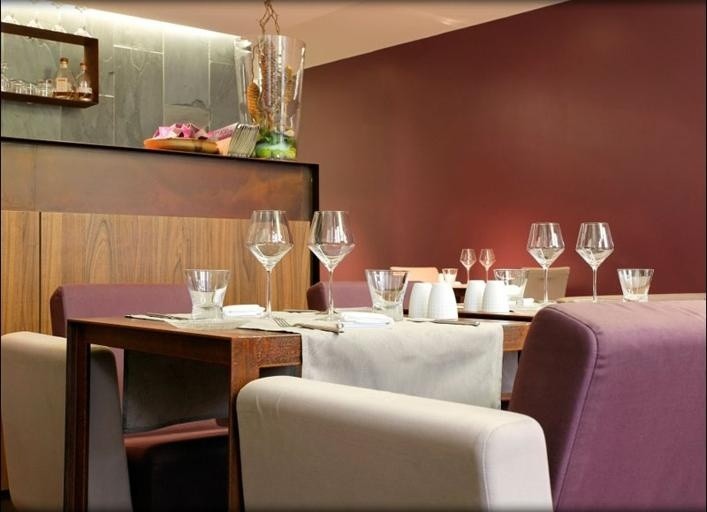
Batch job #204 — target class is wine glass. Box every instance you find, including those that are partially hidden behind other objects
[248,209,294,320]
[307,209,356,320]
[478,249,496,284]
[575,222,614,302]
[527,222,565,308]
[459,248,476,284]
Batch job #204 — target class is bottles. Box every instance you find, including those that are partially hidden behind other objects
[53,57,78,101]
[75,62,92,103]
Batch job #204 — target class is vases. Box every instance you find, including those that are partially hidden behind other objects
[231,34,307,162]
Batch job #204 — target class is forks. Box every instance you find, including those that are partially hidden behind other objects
[272,317,340,334]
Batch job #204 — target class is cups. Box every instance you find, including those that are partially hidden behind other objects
[0,64,53,99]
[365,268,407,321]
[409,267,528,322]
[184,267,230,326]
[615,267,654,304]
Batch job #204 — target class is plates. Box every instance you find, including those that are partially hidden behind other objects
[144,138,220,154]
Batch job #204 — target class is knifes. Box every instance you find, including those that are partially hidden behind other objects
[146,312,191,321]
[409,318,480,327]
